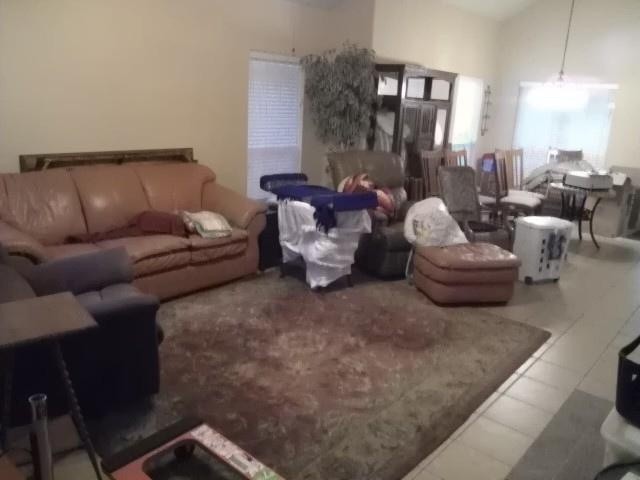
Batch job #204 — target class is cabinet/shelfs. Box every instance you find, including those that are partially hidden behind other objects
[369,64,460,178]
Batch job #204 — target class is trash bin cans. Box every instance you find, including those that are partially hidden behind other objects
[512,215,575,285]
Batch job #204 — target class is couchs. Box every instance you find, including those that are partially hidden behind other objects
[0,160,270,308]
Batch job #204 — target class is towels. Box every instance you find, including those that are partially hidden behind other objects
[66,210,232,244]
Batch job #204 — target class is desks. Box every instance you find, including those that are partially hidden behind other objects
[0,289,106,478]
[277,201,368,291]
[549,180,616,251]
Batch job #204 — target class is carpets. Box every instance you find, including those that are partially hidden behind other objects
[72,262,552,479]
[498,389,616,480]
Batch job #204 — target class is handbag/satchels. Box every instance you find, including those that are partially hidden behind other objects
[185,207,232,239]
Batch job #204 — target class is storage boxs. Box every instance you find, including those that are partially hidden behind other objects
[598,336,640,468]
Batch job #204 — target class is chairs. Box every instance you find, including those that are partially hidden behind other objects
[0,241,164,409]
[321,151,417,283]
[421,147,546,247]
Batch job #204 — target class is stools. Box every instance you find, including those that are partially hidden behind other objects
[412,243,521,308]
